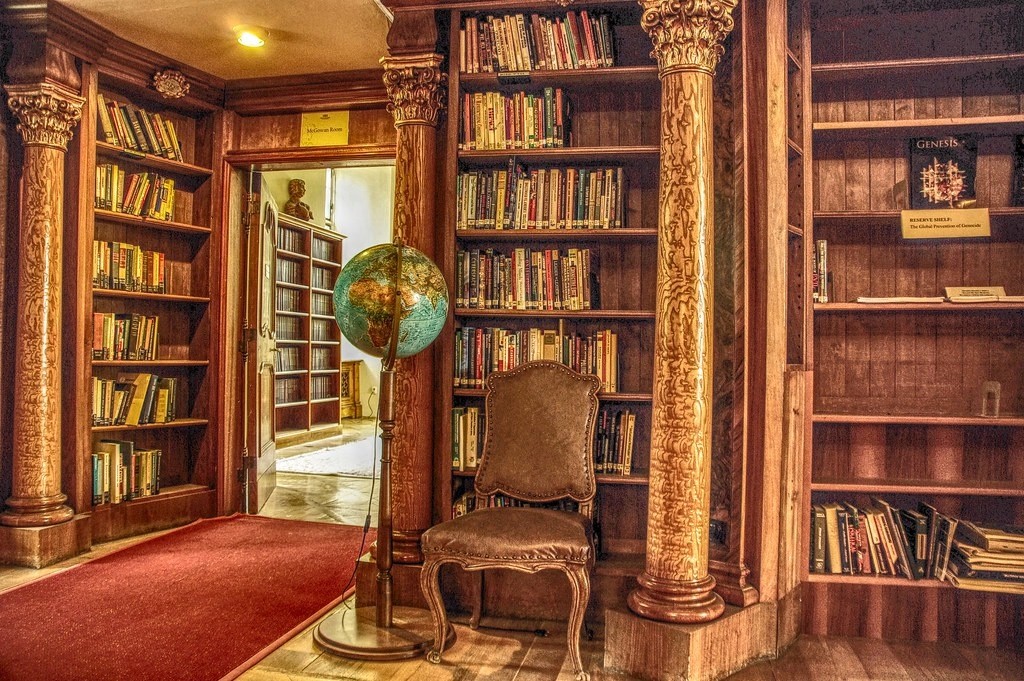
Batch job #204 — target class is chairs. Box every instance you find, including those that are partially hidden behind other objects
[416,359,605,681]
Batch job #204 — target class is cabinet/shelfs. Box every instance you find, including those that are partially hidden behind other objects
[797,1,1022,657]
[439,4,662,563]
[74,21,227,546]
[274,210,349,450]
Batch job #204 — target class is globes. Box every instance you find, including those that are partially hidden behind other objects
[314,237,457,662]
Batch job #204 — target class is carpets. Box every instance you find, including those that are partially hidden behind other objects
[273,433,380,480]
[0,508,377,681]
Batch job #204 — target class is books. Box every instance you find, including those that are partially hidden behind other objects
[806,497,1024,600]
[845,294,1024,303]
[86,87,184,505]
[907,134,980,211]
[815,238,831,304]
[811,241,820,305]
[456,6,640,557]
[267,220,345,412]
